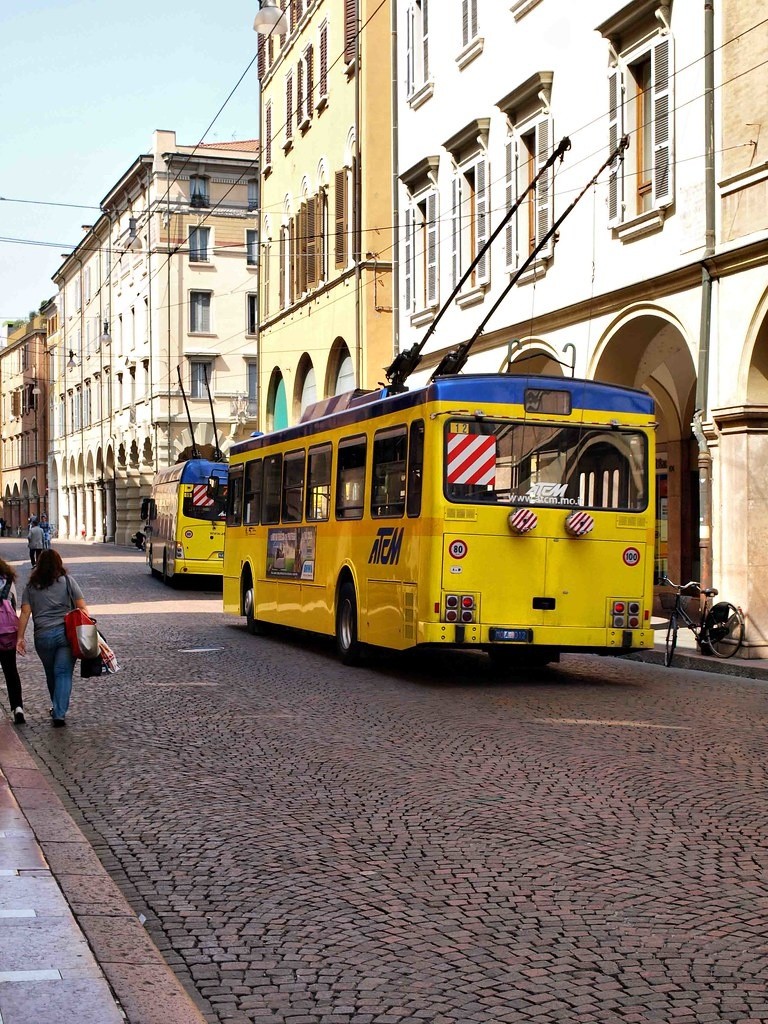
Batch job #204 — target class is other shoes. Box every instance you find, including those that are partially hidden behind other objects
[54,719,66,728]
[50,707,54,717]
[14,706,26,724]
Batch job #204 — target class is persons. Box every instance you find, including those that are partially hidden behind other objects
[131,531,146,552]
[15,548,89,726]
[0,557,25,724]
[0,513,52,569]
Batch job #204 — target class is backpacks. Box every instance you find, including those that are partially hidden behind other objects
[0,577,21,650]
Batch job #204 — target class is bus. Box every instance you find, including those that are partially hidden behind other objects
[141,364,229,592]
[220,138,659,674]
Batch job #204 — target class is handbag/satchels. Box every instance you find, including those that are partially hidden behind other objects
[64,574,102,659]
[80,630,121,678]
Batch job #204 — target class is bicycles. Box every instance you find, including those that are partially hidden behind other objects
[658,576,746,667]
